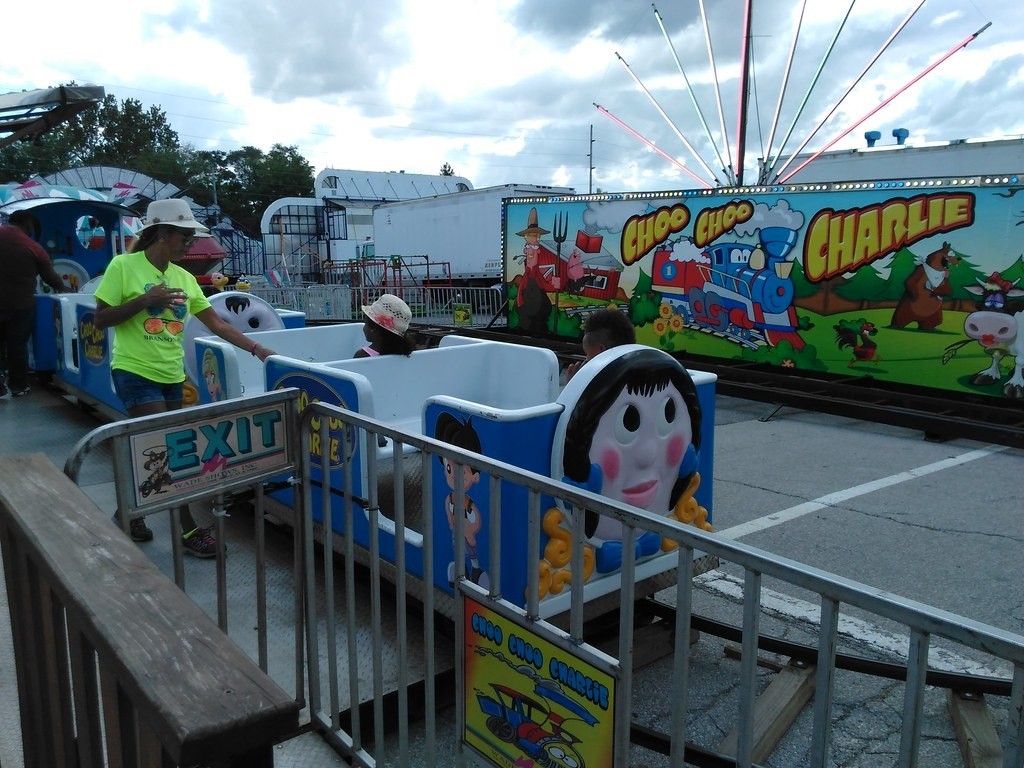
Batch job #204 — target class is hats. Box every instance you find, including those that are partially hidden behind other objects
[361,293,413,339]
[134,198,210,233]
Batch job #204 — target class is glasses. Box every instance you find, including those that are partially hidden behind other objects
[171,228,198,247]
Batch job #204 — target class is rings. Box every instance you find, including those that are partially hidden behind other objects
[170,299,175,305]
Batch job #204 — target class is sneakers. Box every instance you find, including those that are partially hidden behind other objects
[112,508,153,541]
[12,384,33,395]
[0,372,8,396]
[181,526,228,558]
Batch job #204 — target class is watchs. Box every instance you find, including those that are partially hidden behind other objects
[248,342,259,356]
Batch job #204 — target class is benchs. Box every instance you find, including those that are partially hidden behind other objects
[202,313,368,403]
[421,360,719,543]
[318,342,552,507]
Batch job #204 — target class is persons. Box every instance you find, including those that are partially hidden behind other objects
[566,308,638,385]
[352,294,417,360]
[93,197,280,560]
[0,209,77,398]
